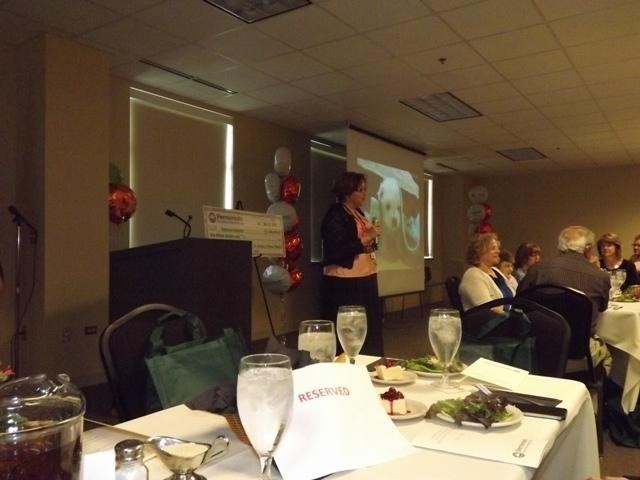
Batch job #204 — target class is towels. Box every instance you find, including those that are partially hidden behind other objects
[184,334,312,414]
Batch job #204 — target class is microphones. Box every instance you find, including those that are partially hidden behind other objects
[165,208,193,239]
[372,216,381,244]
[7,204,38,234]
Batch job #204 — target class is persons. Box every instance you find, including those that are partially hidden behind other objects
[457,222,639,429]
[318,168,384,354]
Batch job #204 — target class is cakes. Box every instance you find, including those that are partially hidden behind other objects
[379,386,407,416]
[376,357,406,381]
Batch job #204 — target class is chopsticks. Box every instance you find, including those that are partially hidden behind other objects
[476,383,492,397]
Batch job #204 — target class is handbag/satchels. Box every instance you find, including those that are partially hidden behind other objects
[604,397,640,448]
[458,338,540,373]
[143,328,251,410]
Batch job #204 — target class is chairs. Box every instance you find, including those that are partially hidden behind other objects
[99,304,208,423]
[445,269,604,458]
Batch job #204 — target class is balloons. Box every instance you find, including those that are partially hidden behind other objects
[105,184,140,225]
[109,162,125,185]
[256,146,305,299]
[466,183,493,234]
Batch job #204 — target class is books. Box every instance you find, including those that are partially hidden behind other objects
[411,415,560,471]
[77,416,161,470]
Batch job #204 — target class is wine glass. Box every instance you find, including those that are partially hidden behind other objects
[335,305,368,366]
[236,352,294,480]
[429,308,462,389]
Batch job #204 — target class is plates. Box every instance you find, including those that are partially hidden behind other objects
[416,362,467,377]
[385,399,428,420]
[436,404,524,428]
[370,371,417,385]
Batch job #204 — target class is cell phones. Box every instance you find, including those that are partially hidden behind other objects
[512,402,567,420]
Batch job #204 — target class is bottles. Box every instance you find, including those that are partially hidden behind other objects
[114,440,149,479]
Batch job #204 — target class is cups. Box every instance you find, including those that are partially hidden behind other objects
[297,320,337,363]
[631,285,640,299]
[608,269,627,299]
[144,434,230,479]
[0,393,87,480]
